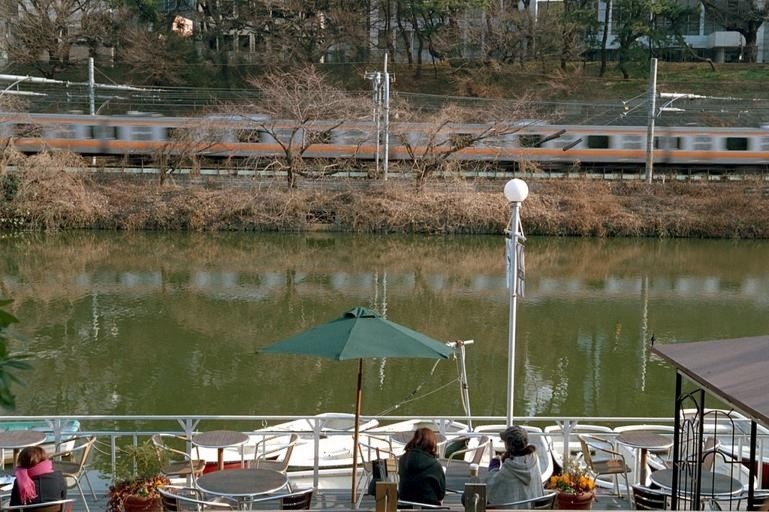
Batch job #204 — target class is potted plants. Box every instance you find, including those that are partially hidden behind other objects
[93,433,178,511]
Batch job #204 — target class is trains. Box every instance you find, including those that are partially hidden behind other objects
[0,107,769,179]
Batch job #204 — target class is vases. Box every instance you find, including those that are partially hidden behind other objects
[554,491,594,510]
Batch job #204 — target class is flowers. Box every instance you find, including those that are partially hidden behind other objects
[548,451,598,492]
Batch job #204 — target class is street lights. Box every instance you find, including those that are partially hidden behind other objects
[500,176,532,426]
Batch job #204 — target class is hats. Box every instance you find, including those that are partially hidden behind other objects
[500,427,528,448]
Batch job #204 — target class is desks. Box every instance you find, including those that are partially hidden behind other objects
[649,469,745,511]
[436,463,490,495]
[191,432,250,472]
[615,432,675,487]
[390,432,448,448]
[0,431,46,476]
[194,468,289,510]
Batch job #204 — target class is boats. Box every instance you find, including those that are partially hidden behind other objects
[183,412,380,476]
[463,424,555,489]
[544,424,653,489]
[275,418,474,490]
[0,419,83,465]
[613,407,769,494]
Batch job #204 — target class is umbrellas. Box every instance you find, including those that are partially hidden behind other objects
[252,304,460,504]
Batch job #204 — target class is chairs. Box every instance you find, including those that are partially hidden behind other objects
[156,485,241,512]
[436,433,490,504]
[246,433,298,509]
[708,498,722,511]
[577,434,634,511]
[486,489,557,510]
[736,489,768,511]
[152,432,207,487]
[2,498,77,512]
[665,434,720,511]
[352,432,399,510]
[42,433,97,511]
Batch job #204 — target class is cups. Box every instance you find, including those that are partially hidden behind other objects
[468,463,479,480]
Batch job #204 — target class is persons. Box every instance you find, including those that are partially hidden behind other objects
[398,425,447,511]
[8,445,67,512]
[461,425,546,509]
